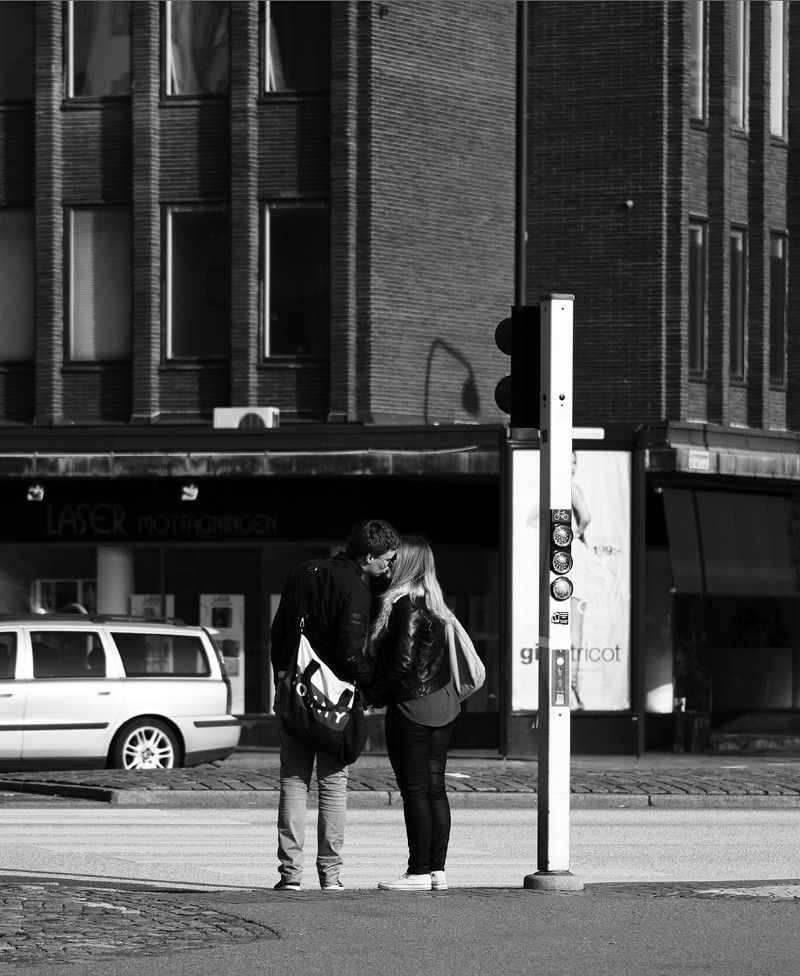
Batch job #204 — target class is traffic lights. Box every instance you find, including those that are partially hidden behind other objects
[492,302,538,430]
[551,509,574,601]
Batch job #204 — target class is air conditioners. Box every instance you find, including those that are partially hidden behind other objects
[214,407,280,428]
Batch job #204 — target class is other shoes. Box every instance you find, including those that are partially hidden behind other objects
[322,880,345,890]
[378,873,432,891]
[430,871,447,890]
[274,879,300,890]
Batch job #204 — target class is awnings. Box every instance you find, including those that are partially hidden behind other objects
[654,486,800,599]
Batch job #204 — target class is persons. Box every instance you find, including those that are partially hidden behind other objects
[269,521,402,889]
[570,452,592,708]
[364,536,461,891]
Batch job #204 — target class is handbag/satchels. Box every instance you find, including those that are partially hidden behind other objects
[279,634,368,766]
[445,618,486,702]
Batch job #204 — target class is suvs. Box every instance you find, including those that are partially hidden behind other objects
[1,614,241,772]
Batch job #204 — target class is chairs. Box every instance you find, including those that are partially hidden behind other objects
[87,648,103,675]
